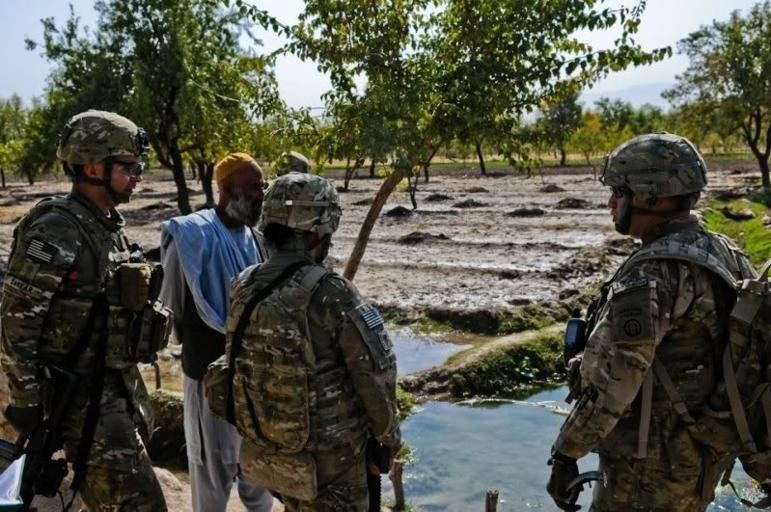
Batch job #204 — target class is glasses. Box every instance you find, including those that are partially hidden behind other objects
[112,159,142,176]
[613,187,631,198]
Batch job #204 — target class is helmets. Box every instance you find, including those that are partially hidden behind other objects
[275,150,308,174]
[56,110,145,166]
[600,132,708,201]
[261,171,342,238]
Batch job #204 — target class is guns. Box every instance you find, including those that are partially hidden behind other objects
[365,438,391,512]
[20,360,82,512]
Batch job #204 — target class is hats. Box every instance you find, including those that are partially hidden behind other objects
[216,153,254,183]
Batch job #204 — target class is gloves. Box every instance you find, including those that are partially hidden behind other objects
[548,453,585,511]
[5,406,43,438]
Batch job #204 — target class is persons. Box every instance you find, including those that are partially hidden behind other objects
[158,153,274,512]
[0,109,171,512]
[206,171,401,512]
[272,150,309,178]
[546,134,760,512]
